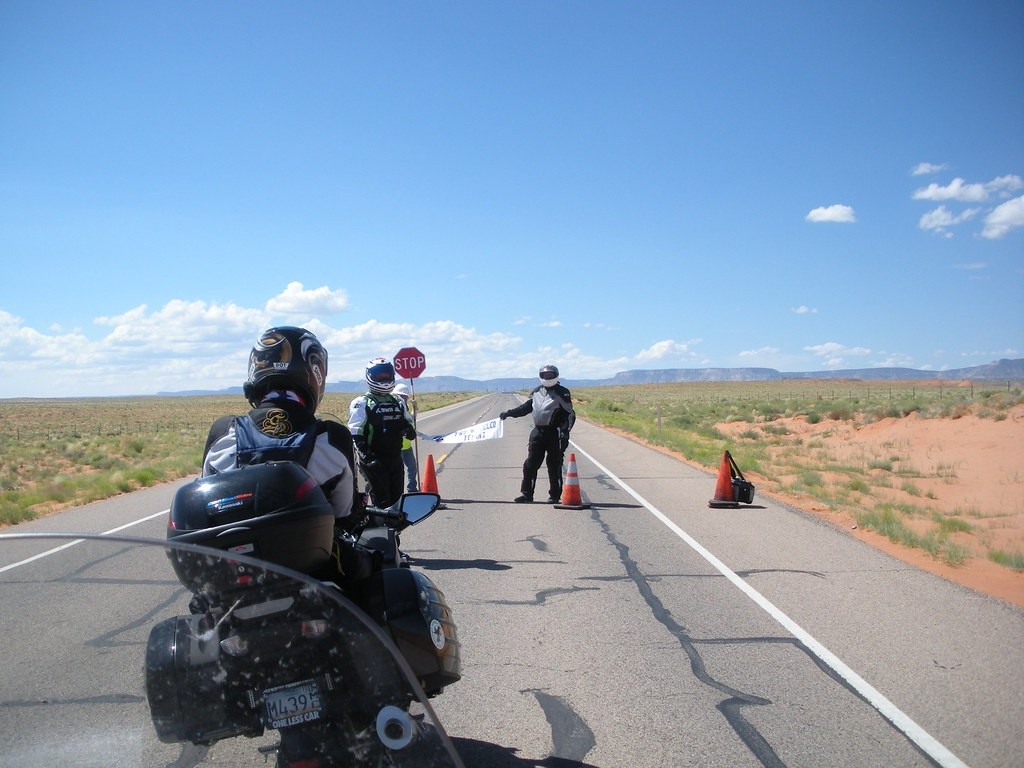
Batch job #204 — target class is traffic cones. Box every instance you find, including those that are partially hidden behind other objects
[422,454,447,510]
[552,453,591,511]
[707,449,740,508]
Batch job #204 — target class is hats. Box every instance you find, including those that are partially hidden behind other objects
[391,384,410,397]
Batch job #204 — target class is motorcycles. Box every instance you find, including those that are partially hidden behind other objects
[141,413,463,768]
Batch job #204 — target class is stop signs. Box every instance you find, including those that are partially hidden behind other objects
[393,347,426,379]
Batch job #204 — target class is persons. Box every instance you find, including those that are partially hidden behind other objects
[500,365,576,504]
[189,327,386,615]
[347,358,418,563]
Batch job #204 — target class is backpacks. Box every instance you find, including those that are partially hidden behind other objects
[235,415,322,469]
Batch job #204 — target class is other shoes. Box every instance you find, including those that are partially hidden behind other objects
[514,496,533,504]
[547,496,559,503]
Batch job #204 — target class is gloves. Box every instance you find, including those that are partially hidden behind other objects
[364,458,382,486]
[499,411,509,420]
[432,435,443,441]
[406,427,416,440]
[561,438,569,452]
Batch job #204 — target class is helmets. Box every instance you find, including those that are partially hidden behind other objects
[243,326,328,415]
[539,365,559,387]
[365,358,396,395]
